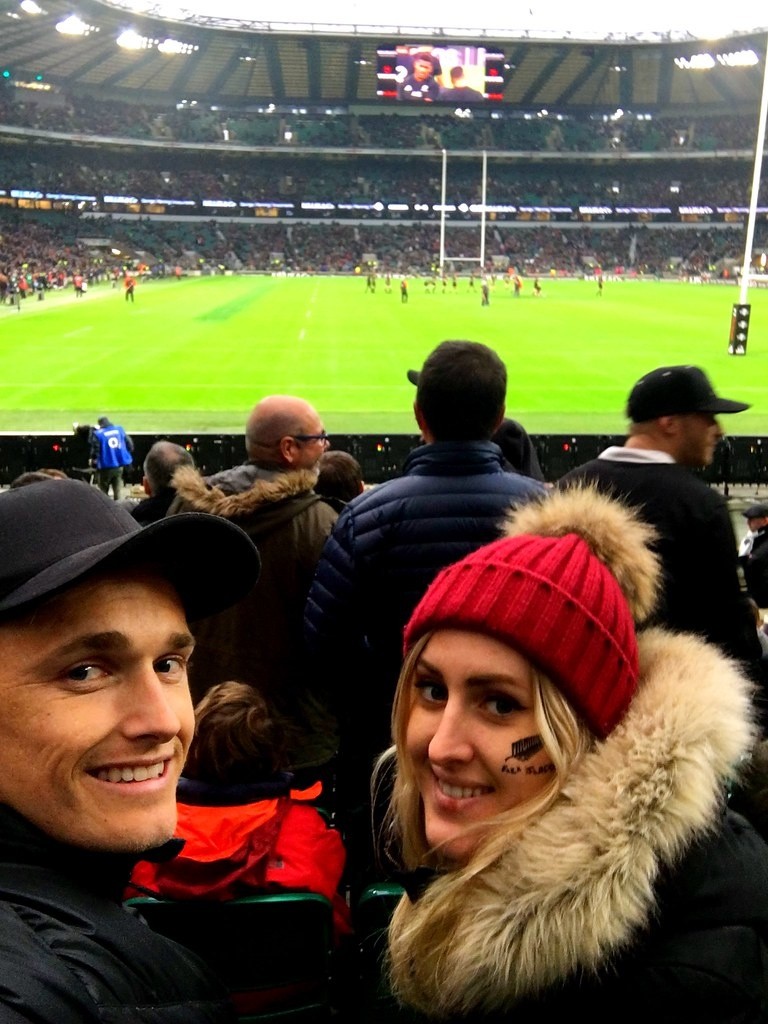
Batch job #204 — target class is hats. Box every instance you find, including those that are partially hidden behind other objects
[0,480,261,623]
[742,506,768,519]
[627,366,748,417]
[401,475,666,739]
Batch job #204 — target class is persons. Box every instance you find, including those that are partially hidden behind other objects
[401,281,408,302]
[0,340,768,905]
[124,272,136,301]
[0,68,767,304]
[373,479,768,1024]
[0,481,261,1024]
[400,55,485,101]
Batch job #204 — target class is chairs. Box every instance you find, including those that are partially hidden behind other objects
[121,881,433,1024]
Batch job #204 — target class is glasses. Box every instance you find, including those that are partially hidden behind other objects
[247,430,328,449]
